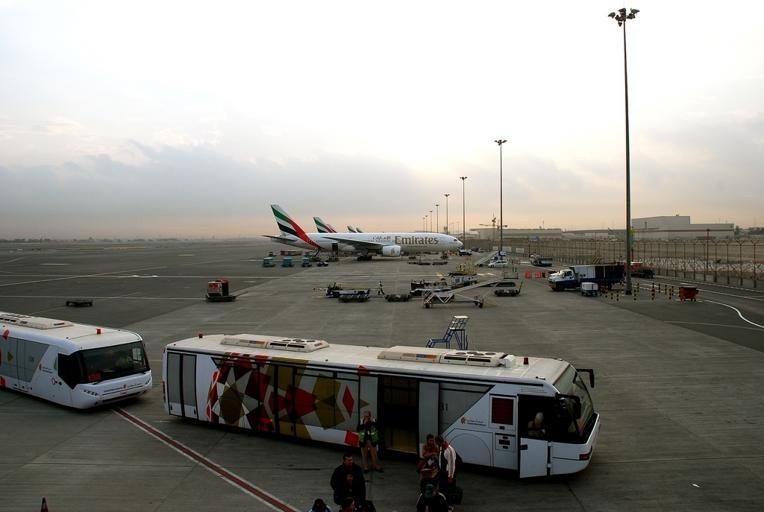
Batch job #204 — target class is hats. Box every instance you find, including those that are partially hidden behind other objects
[424,483,435,497]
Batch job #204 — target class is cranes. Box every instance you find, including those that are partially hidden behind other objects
[478,218,508,232]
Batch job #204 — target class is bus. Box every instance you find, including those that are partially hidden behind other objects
[0,304,156,420]
[159,328,606,482]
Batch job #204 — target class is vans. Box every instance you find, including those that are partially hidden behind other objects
[458,250,472,257]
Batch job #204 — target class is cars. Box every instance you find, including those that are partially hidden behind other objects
[486,259,507,268]
[471,246,485,254]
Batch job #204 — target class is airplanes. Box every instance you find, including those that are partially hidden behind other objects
[258,203,463,262]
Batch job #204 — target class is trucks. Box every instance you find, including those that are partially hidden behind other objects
[546,263,625,292]
[623,258,654,279]
[529,252,553,267]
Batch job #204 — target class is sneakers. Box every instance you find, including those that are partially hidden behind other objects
[364,467,385,473]
[447,505,456,512]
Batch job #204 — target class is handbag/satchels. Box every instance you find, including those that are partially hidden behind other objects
[438,483,464,505]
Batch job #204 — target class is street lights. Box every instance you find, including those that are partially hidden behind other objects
[706,227,711,276]
[604,4,644,300]
[492,138,510,252]
[458,175,470,249]
[443,192,452,235]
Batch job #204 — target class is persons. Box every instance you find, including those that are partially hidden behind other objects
[338,497,355,511]
[356,411,384,471]
[308,498,332,511]
[417,432,465,511]
[330,452,366,508]
